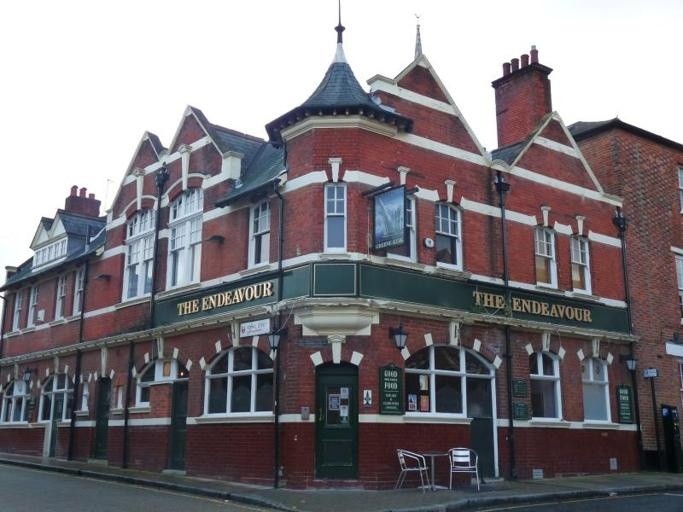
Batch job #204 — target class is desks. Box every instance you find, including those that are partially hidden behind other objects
[417,450,451,492]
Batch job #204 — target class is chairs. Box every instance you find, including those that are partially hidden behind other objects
[448,446,480,494]
[392,448,433,496]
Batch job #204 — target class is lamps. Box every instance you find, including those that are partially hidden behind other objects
[389,322,408,352]
[96,273,112,281]
[620,354,638,376]
[24,367,38,385]
[268,326,288,352]
[206,234,226,244]
